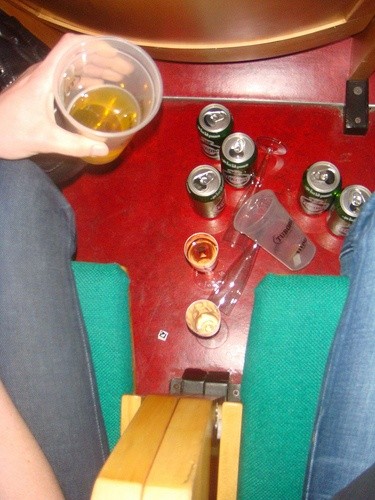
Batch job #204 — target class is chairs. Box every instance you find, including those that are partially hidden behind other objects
[68,259,351,500]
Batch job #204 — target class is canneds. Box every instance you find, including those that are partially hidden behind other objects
[219,132,257,188]
[185,165,225,219]
[327,184,372,237]
[197,103,234,160]
[298,161,341,216]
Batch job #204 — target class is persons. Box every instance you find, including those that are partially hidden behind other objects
[0,30,133,500]
[302,192,375,500]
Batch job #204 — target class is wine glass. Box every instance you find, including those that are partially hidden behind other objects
[203,136,287,315]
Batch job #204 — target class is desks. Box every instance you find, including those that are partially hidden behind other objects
[0,0,375,84]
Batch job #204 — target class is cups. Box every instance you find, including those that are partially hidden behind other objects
[233,189,317,271]
[183,232,218,272]
[54,36,163,165]
[185,299,221,337]
